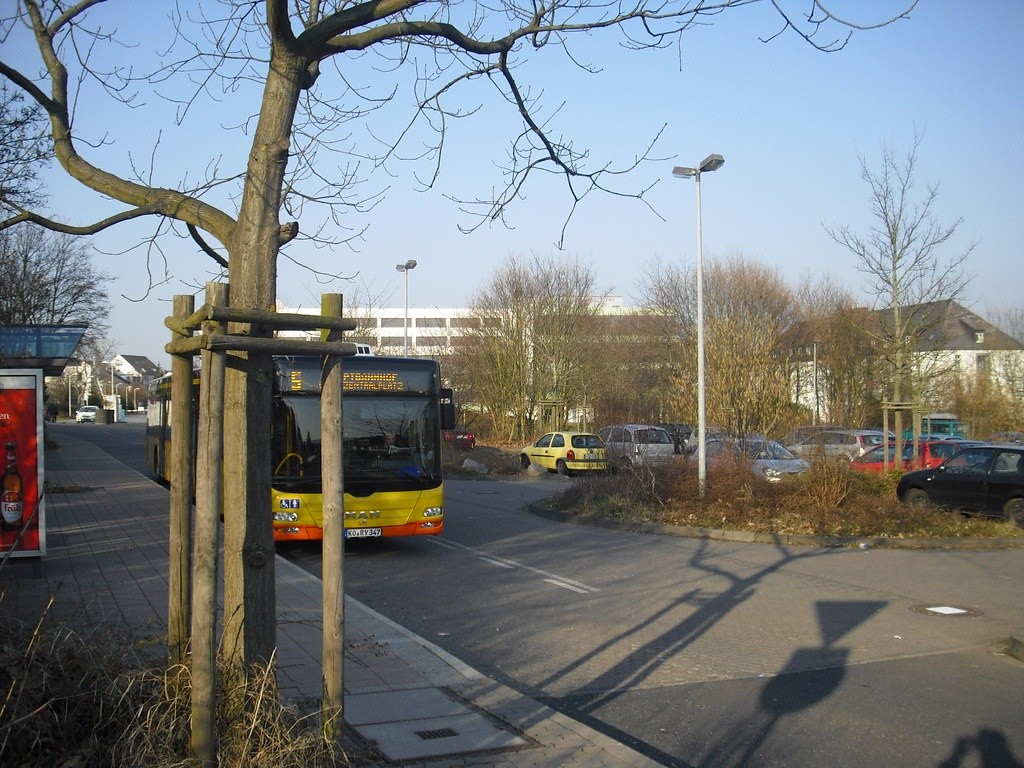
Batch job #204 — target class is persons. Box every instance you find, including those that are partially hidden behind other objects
[371,429,403,457]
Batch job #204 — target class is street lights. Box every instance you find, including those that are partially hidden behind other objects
[101,359,140,412]
[69,373,79,416]
[396,260,417,357]
[672,154,725,498]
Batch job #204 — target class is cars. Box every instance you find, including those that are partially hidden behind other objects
[659,427,1024,529]
[519,431,609,479]
[75,405,101,424]
[440,425,476,450]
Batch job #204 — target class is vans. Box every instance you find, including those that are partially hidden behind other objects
[590,424,675,476]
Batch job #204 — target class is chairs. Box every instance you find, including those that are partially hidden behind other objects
[1008,458,1024,477]
[575,438,584,448]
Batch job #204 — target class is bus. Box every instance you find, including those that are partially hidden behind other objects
[148,342,455,552]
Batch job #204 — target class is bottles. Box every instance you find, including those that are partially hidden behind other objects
[0,441,23,531]
